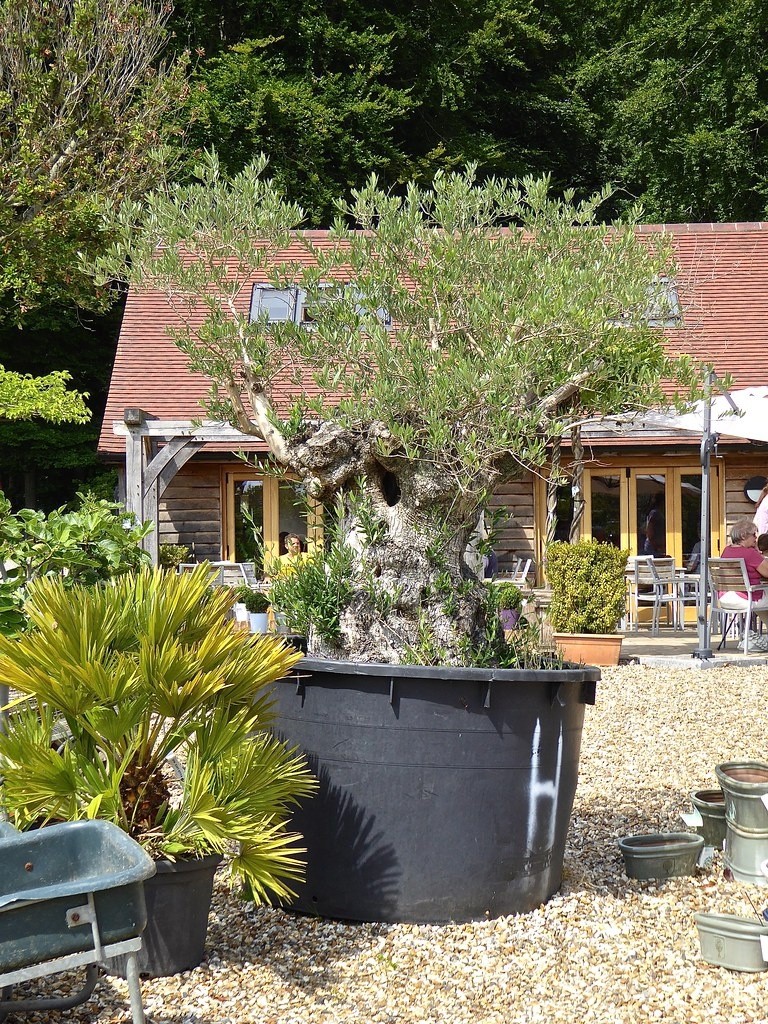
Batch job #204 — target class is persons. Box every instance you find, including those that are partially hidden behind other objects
[685,523,701,574]
[717,485,768,653]
[278,533,318,580]
[643,492,665,558]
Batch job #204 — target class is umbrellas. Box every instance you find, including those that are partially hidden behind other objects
[602,386,768,442]
[591,474,701,516]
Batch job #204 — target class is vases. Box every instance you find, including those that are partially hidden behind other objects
[623,833,705,881]
[717,821,768,877]
[694,914,768,969]
[0,816,153,975]
[691,789,726,852]
[713,761,768,833]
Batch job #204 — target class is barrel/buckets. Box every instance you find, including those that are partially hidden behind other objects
[620,759,768,974]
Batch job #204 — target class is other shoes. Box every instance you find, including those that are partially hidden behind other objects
[737,640,765,652]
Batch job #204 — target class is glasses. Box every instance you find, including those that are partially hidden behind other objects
[750,532,756,537]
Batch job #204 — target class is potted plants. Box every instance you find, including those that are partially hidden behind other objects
[233,586,254,631]
[498,586,523,631]
[546,538,631,666]
[274,583,301,635]
[0,566,323,981]
[245,592,270,633]
[97,154,726,929]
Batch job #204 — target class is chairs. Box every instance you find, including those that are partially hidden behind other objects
[646,557,699,637]
[707,558,768,656]
[634,559,677,635]
